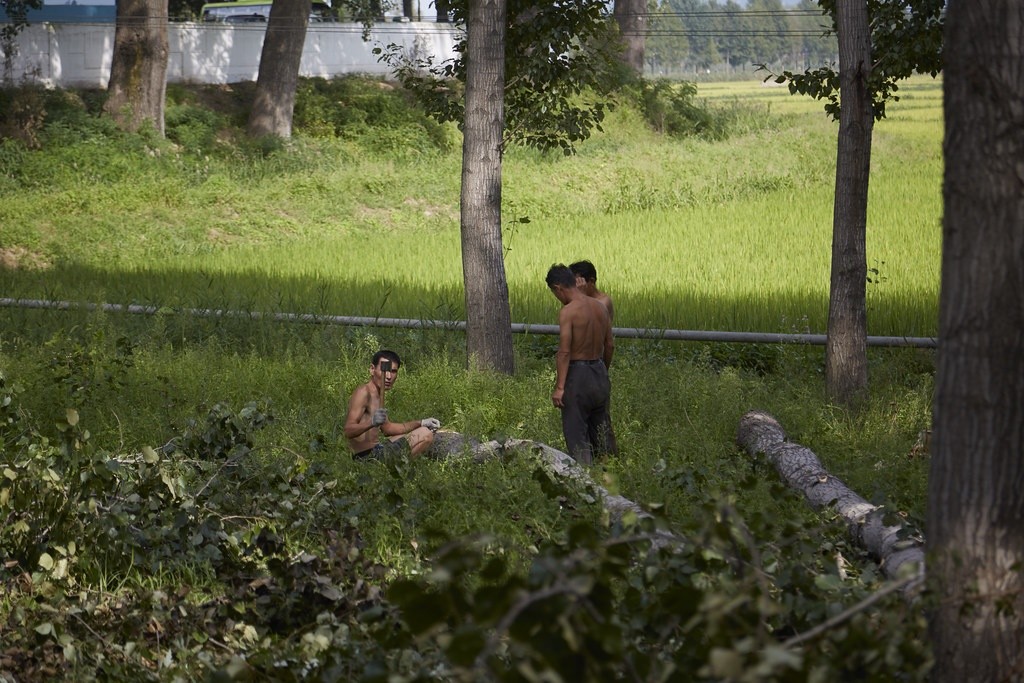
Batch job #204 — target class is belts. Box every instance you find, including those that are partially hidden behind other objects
[568,358,603,366]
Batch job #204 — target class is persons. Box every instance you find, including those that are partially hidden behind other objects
[545,261,620,471]
[343,349,440,480]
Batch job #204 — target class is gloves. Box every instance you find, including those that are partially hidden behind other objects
[422,418,440,429]
[371,408,387,426]
